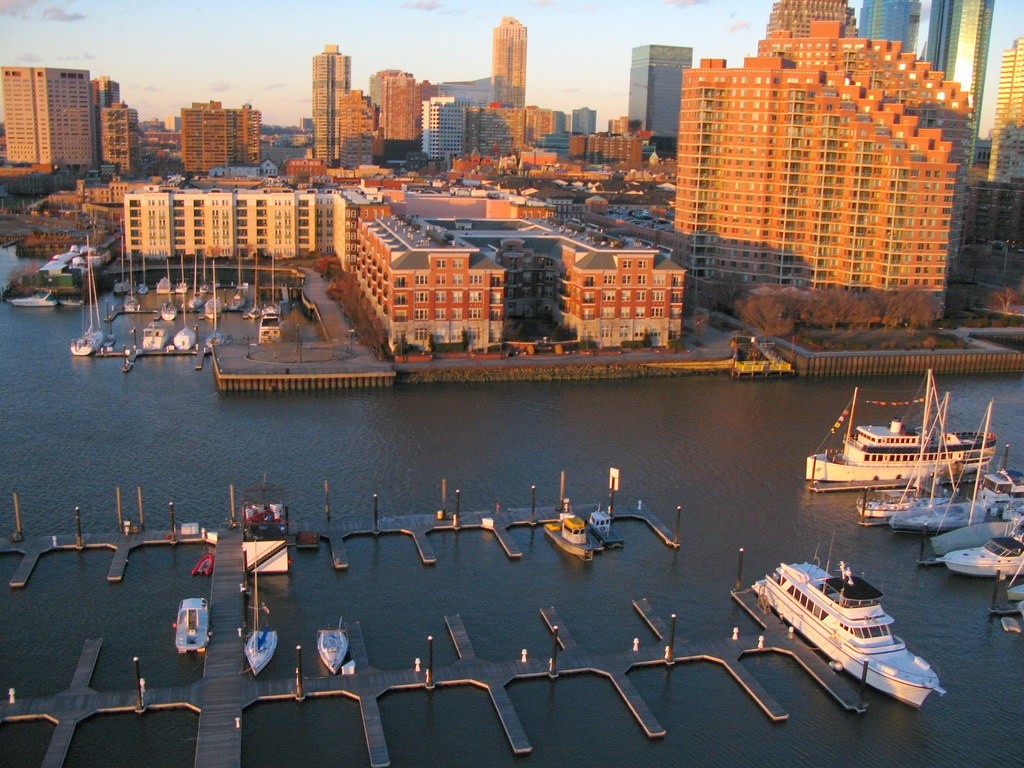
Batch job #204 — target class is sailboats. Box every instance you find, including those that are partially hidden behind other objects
[807,367,1024,608]
[112,234,279,323]
[173,251,197,350]
[244,537,279,674]
[206,259,227,349]
[70,232,103,355]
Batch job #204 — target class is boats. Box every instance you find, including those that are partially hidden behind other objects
[749,526,947,709]
[171,596,211,654]
[141,320,169,352]
[56,295,85,306]
[542,510,605,560]
[6,288,60,307]
[259,314,282,345]
[190,552,214,578]
[317,627,349,672]
